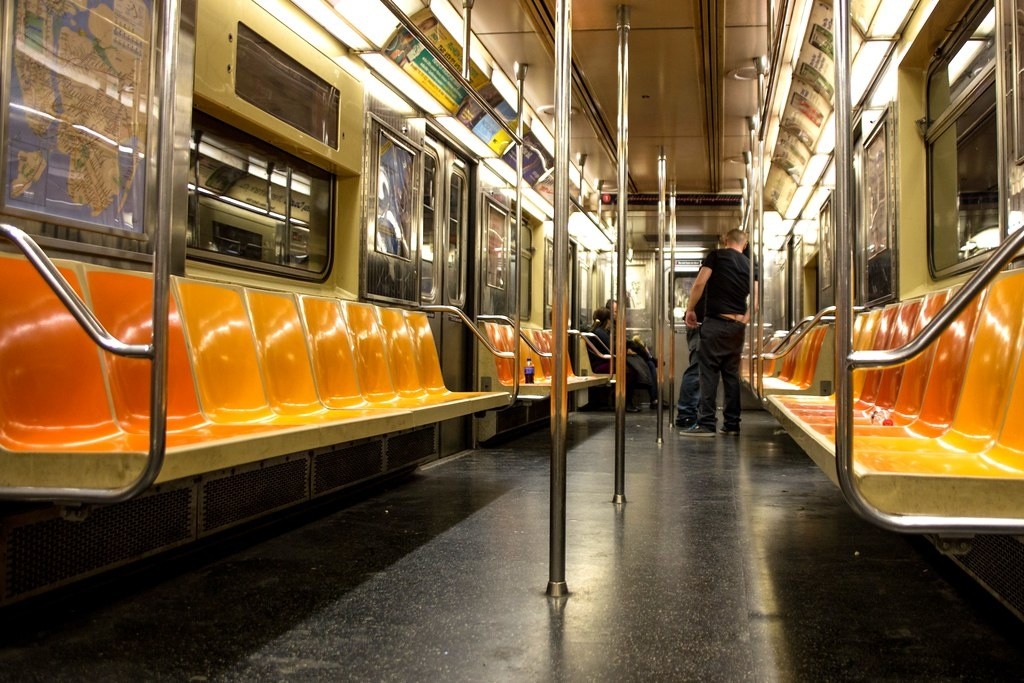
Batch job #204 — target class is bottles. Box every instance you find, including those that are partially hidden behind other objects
[868,406,893,426]
[524,358,535,383]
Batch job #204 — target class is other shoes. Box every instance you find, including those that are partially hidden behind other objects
[627,403,641,413]
[675,415,696,427]
[680,422,714,437]
[650,397,676,409]
[719,426,739,436]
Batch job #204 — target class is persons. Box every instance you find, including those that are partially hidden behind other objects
[675,242,756,427]
[679,229,758,437]
[385,16,438,67]
[587,300,675,411]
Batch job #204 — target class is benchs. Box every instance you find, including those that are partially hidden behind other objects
[739,228,1024,535]
[0,225,616,502]
